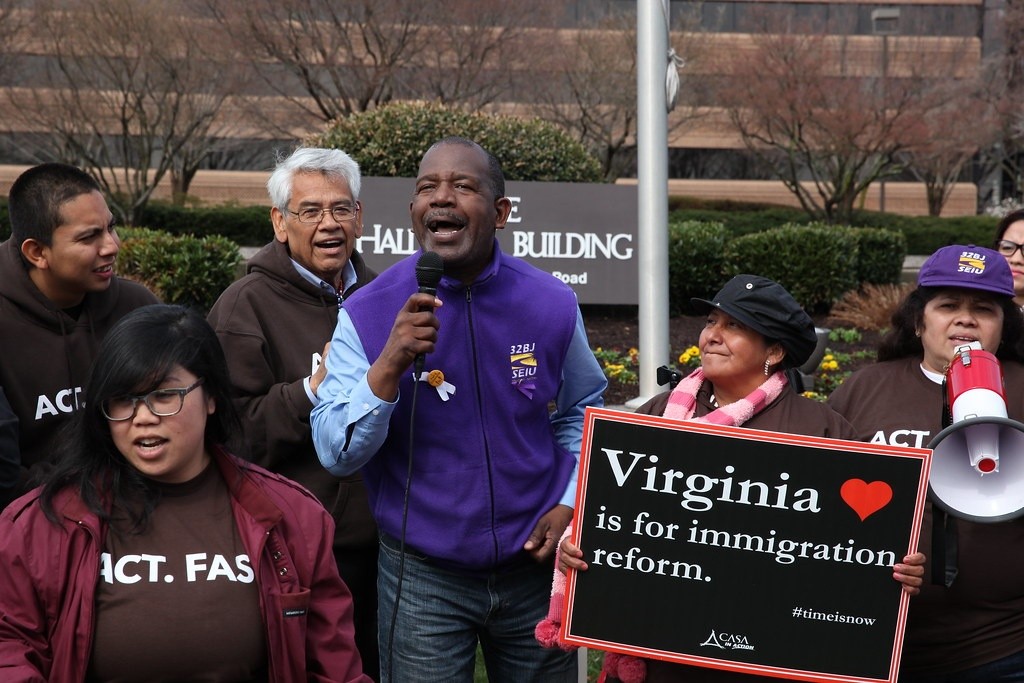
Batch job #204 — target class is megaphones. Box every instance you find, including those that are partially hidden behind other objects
[926,348,1024,522]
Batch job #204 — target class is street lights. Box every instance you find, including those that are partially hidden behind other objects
[870,8,901,215]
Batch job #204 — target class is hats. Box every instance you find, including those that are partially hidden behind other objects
[691,273,819,370]
[917,244,1016,298]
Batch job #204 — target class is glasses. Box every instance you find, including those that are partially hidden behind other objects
[285,198,357,223]
[996,240,1024,258]
[100,380,205,422]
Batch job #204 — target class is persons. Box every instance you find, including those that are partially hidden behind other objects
[557,210,1024,683]
[0,159,372,683]
[206,136,611,683]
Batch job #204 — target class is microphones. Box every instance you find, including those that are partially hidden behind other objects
[414,251,444,378]
